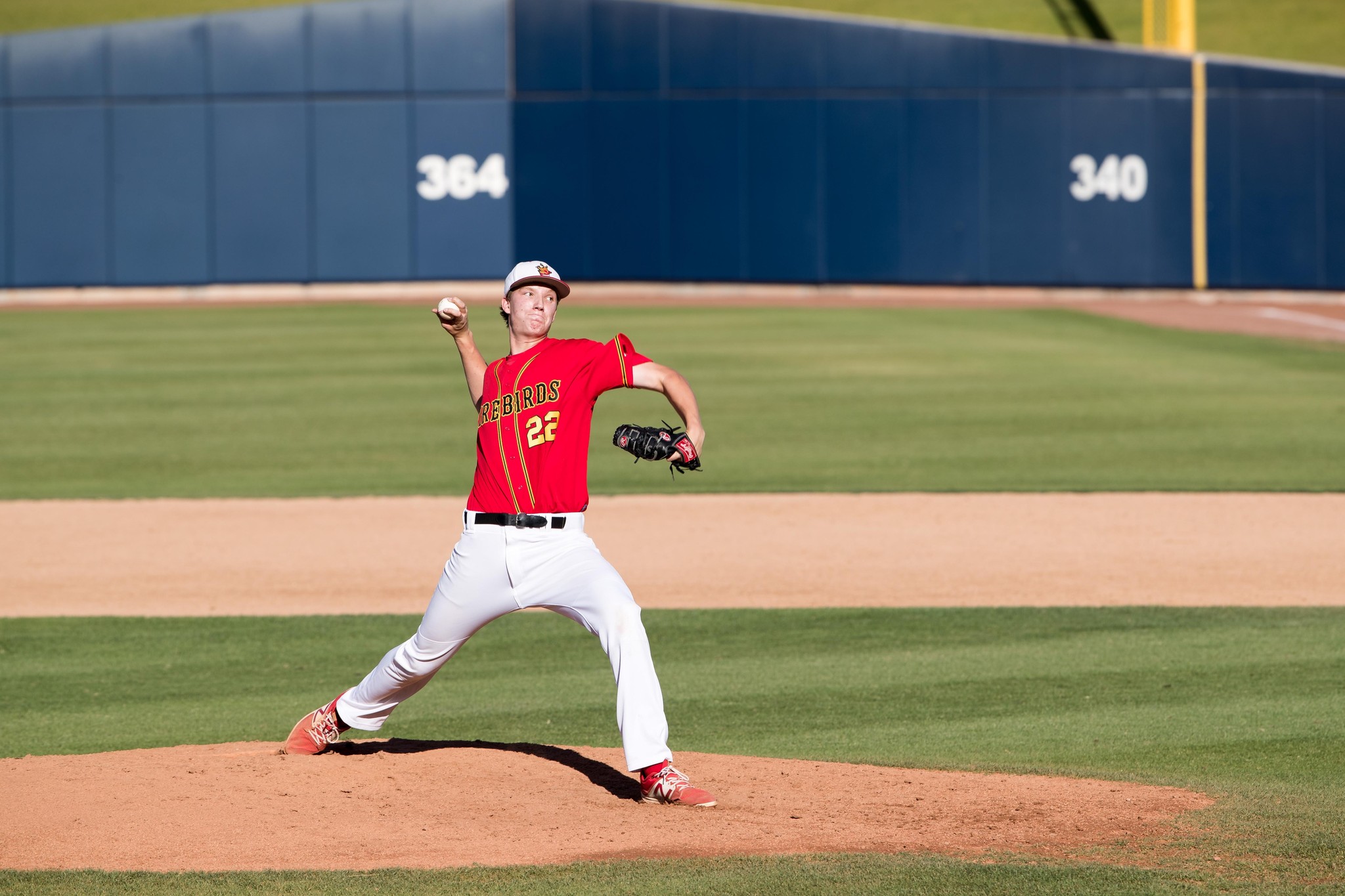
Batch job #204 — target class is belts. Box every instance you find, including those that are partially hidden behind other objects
[464,511,566,530]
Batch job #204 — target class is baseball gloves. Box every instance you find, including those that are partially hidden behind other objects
[612,424,702,471]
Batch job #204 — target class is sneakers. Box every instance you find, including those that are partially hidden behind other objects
[640,764,718,809]
[284,686,359,755]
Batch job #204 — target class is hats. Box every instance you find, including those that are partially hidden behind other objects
[504,261,571,301]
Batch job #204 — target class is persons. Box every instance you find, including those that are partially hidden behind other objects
[282,260,716,807]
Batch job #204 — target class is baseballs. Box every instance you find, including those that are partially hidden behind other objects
[438,298,460,320]
